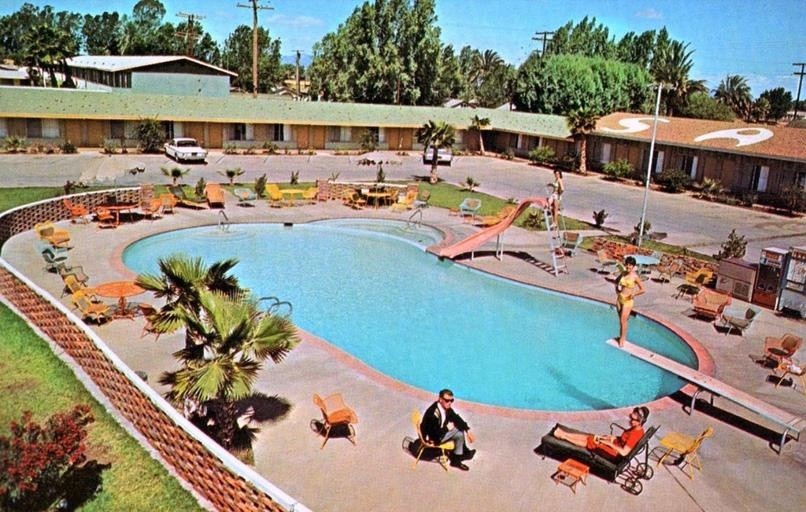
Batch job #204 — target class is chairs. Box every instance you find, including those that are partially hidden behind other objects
[764,331,806,395]
[598,244,705,283]
[554,232,582,257]
[673,267,762,334]
[64,181,430,229]
[30,223,110,326]
[542,422,713,494]
[449,198,513,227]
[313,392,468,473]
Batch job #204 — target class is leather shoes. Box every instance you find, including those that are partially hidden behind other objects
[449,448,477,471]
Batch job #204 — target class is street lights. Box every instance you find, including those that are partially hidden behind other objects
[636,80,664,247]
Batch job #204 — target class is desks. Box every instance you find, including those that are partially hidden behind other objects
[96,280,146,320]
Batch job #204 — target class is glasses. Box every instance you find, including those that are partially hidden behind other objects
[441,397,454,403]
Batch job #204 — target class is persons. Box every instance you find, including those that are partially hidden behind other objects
[553,407,650,465]
[548,170,564,228]
[420,390,477,472]
[615,256,645,348]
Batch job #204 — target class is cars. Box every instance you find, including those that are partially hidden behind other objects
[163,137,206,163]
[423,142,451,165]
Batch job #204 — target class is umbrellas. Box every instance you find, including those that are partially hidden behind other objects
[355,150,402,183]
